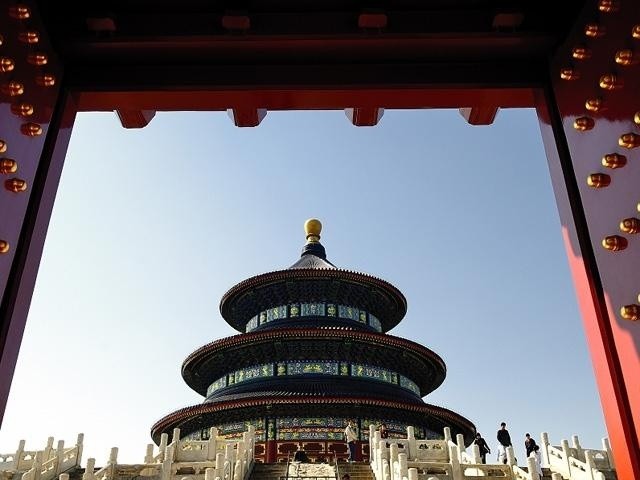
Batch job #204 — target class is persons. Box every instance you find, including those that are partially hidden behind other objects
[474,422,543,480]
[345,418,359,463]
[379,423,388,438]
